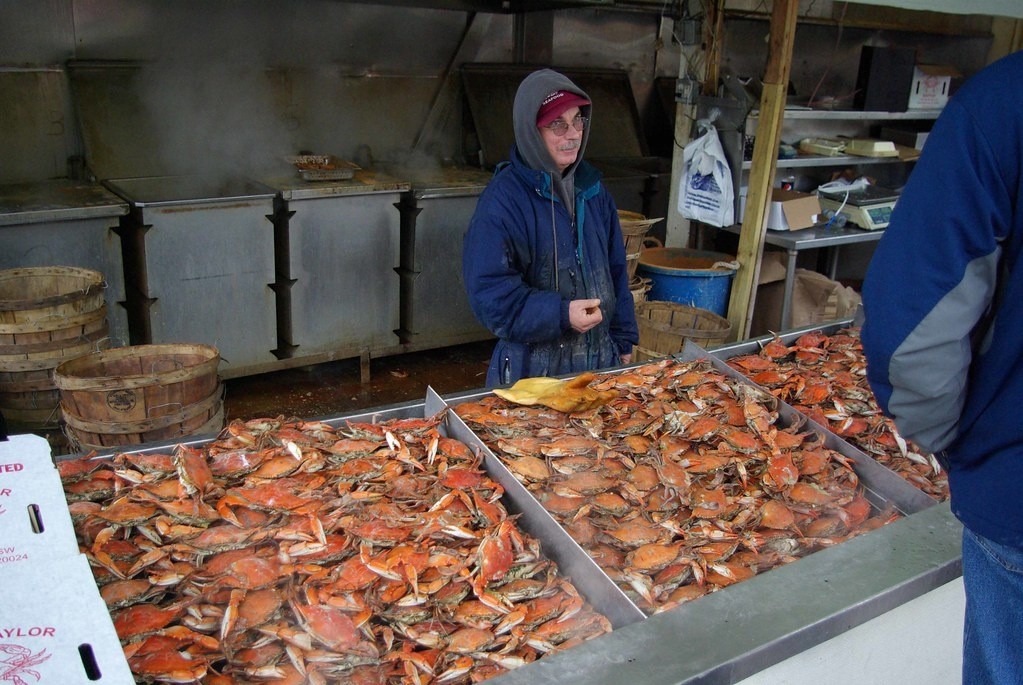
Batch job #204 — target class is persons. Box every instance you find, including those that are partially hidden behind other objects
[861,50,1023,685]
[463,70,640,390]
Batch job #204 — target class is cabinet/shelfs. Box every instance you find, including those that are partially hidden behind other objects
[665,77,947,331]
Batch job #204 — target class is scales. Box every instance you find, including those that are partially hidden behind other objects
[818,185,902,231]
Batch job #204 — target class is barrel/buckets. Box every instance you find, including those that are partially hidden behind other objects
[618,210,736,363]
[0,265,230,454]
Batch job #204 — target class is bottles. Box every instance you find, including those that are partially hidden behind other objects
[781,167,795,191]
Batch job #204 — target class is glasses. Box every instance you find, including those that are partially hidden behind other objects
[544,117,588,136]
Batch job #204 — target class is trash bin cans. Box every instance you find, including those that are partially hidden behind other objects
[635,247,738,319]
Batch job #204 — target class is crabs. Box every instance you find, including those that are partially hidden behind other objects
[55,322,950,685]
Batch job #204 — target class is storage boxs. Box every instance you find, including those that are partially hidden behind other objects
[0,433,136,685]
[740,187,822,232]
[749,251,863,339]
[881,125,932,151]
[893,63,963,109]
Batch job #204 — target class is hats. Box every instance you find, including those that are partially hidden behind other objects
[536,90,591,127]
